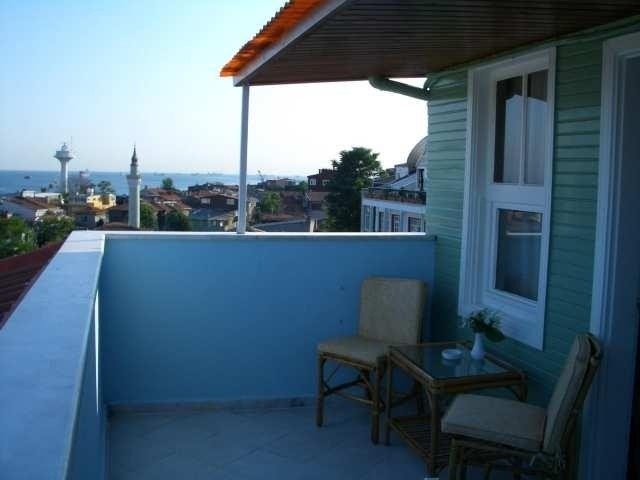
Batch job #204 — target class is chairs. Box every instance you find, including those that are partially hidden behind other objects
[316,279,426,446]
[440,334,603,473]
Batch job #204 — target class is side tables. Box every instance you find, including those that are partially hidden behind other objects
[385,343,530,476]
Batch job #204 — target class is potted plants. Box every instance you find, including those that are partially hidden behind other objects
[469,308,506,361]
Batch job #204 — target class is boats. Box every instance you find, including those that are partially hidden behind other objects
[79,168,91,177]
[23,175,33,179]
